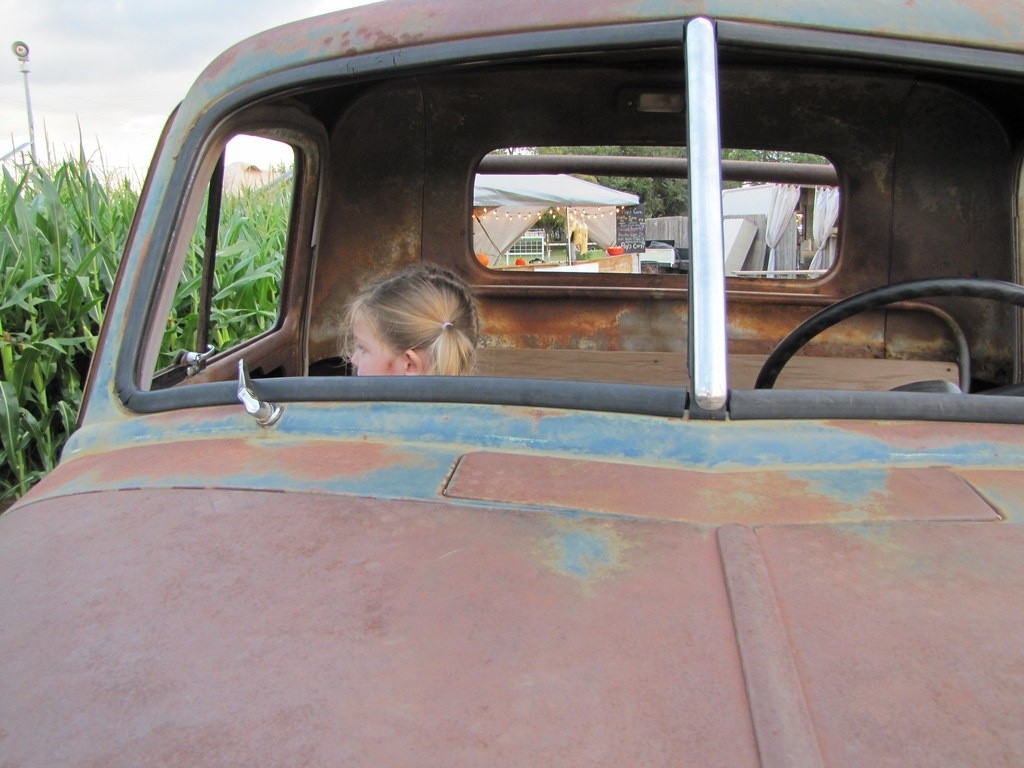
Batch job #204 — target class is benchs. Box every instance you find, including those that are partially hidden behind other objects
[469,283,969,395]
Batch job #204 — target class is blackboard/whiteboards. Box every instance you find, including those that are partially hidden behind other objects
[615,204,645,254]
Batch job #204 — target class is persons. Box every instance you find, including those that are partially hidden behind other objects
[351,262,481,376]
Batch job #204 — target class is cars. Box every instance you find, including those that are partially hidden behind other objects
[0,0,1024,768]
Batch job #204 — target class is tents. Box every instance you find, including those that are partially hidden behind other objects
[471,172,640,268]
